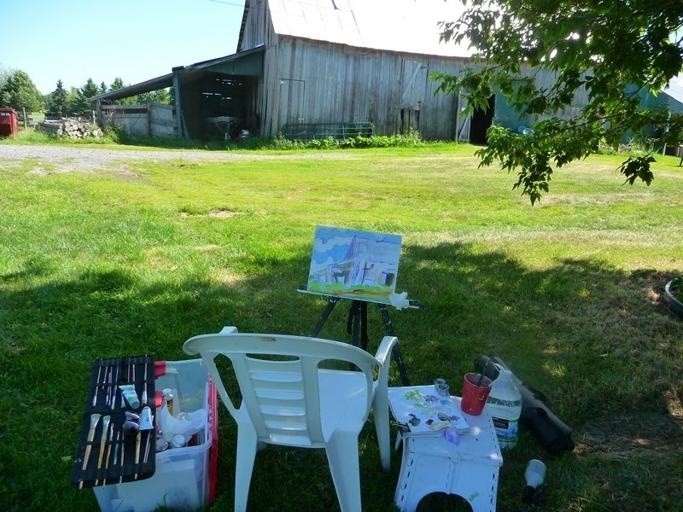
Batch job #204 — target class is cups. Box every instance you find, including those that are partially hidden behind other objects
[459,371,495,416]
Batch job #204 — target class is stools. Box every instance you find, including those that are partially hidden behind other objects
[392,399,502,512]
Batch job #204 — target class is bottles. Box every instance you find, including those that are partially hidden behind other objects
[520,457,548,504]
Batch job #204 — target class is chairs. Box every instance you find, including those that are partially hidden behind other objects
[182,324,398,512]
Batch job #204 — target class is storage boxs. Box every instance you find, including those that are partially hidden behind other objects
[90,354,217,512]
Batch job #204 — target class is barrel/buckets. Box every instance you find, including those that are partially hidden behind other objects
[486,362,525,453]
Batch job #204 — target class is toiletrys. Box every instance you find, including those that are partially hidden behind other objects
[119,385,139,409]
[139,406,154,432]
